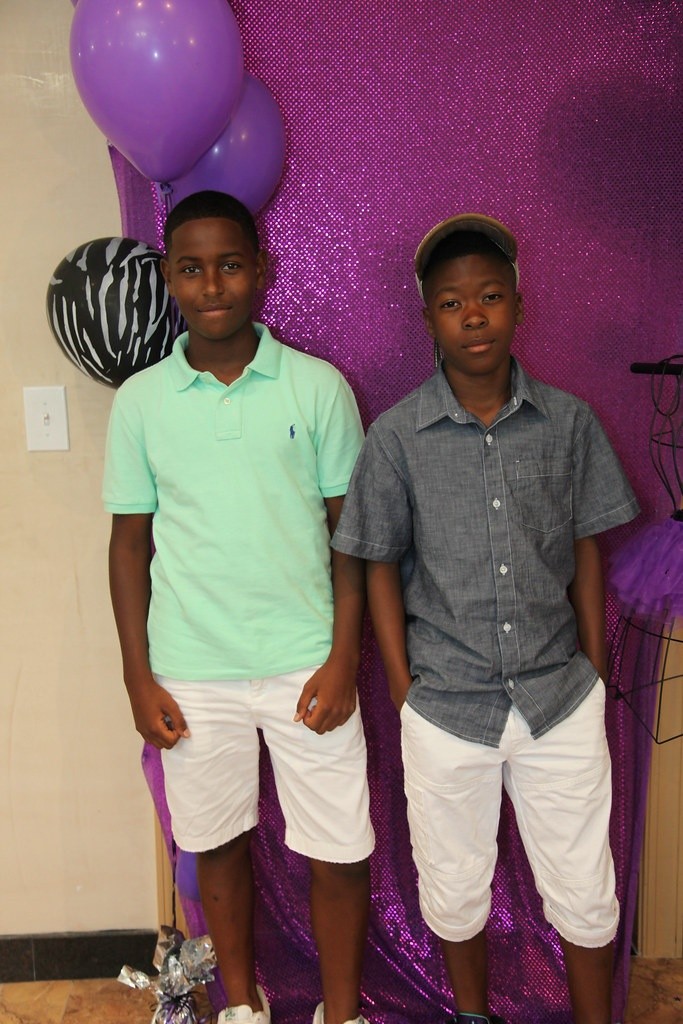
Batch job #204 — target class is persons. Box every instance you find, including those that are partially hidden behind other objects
[100,190,374,1024]
[329,211,640,1024]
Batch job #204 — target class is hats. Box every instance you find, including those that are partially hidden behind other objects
[413,213,519,307]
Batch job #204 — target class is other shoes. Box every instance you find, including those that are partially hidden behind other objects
[217,984,271,1024]
[312,1002,368,1024]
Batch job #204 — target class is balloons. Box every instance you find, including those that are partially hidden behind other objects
[71,0,243,183]
[156,73,286,215]
[46,236,173,390]
